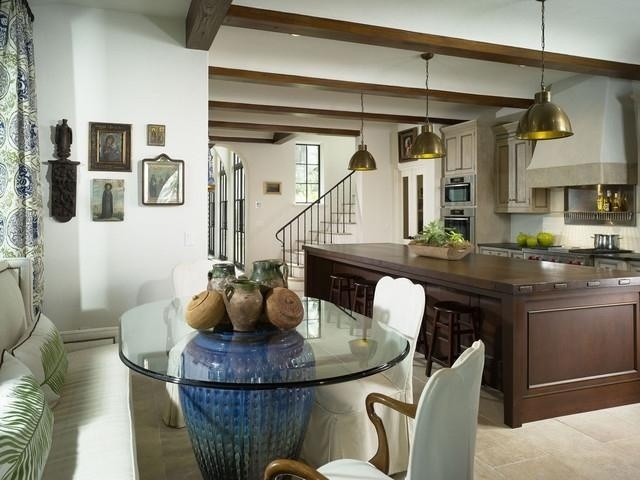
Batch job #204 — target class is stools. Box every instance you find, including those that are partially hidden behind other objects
[326,273,358,328]
[349,280,378,340]
[426,301,483,377]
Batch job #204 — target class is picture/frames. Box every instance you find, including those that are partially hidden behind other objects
[91,123,131,171]
[92,179,124,222]
[397,127,418,162]
[142,154,184,205]
[148,124,165,146]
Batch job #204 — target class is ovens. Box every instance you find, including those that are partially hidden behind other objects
[440,173,476,245]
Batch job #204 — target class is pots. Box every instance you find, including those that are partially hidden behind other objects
[594,233,621,246]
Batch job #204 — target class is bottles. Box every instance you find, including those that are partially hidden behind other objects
[603,190,612,212]
[596,184,604,211]
[612,192,620,211]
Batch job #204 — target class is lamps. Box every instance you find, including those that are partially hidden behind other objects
[516,0,574,140]
[348,94,377,171]
[408,54,445,159]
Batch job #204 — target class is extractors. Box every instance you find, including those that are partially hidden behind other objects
[527,93,640,188]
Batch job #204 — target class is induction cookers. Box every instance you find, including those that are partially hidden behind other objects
[569,248,635,254]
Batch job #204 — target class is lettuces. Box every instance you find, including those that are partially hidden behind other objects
[408,220,470,248]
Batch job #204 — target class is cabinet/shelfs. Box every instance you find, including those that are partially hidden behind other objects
[492,121,550,214]
[399,157,440,244]
[440,119,511,247]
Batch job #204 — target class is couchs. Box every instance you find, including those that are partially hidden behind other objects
[1,257,141,480]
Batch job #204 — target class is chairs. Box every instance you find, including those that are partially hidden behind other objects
[166,261,237,429]
[299,276,426,477]
[263,340,485,479]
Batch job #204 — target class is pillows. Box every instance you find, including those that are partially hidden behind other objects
[1,349,55,480]
[9,310,69,408]
